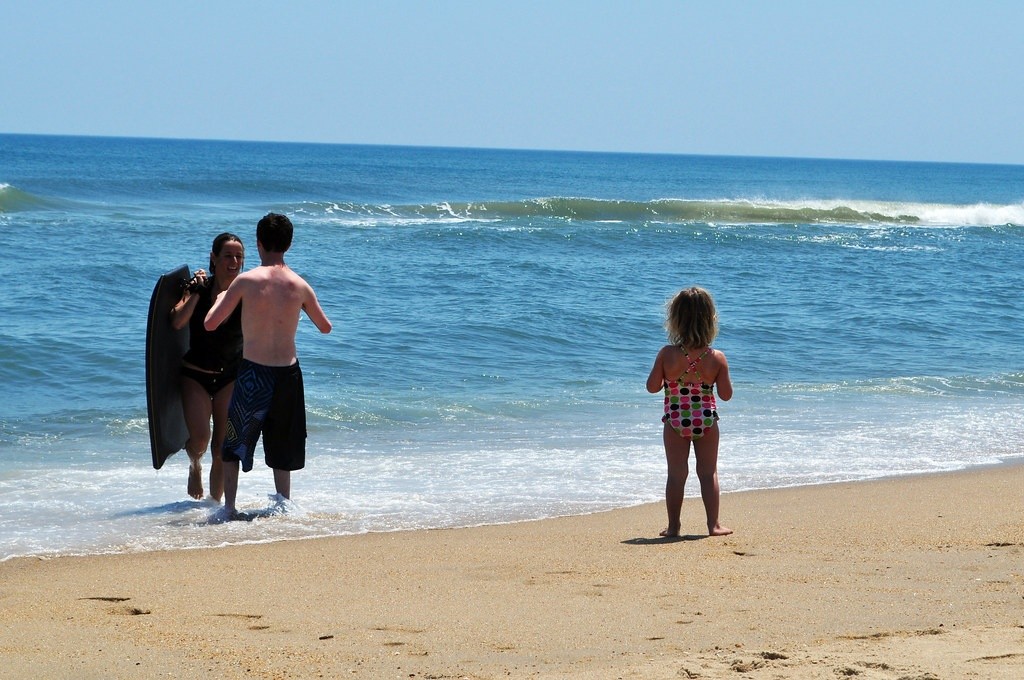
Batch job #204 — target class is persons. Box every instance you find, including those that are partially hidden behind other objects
[203,213,332,508]
[168,232,245,502]
[646,287,736,537]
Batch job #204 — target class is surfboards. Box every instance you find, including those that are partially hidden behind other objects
[145,262,190,472]
